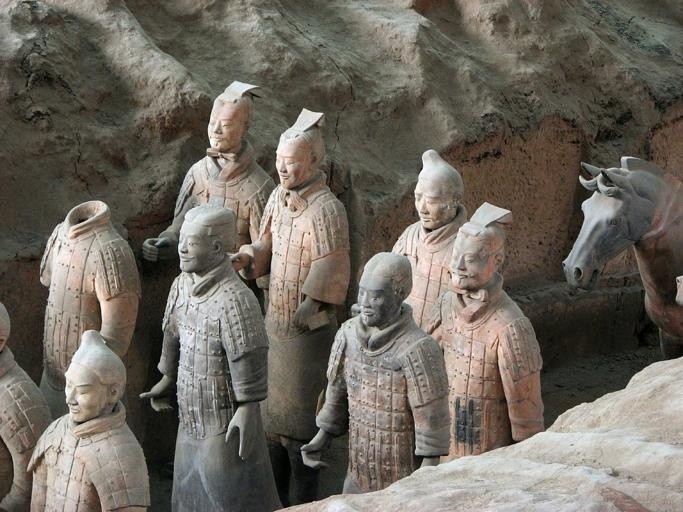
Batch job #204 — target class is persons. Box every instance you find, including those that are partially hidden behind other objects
[39,200,142,420]
[142,80,277,313]
[140,204,283,512]
[0,302,51,512]
[226,108,351,507]
[27,329,150,512]
[424,202,545,464]
[298,251,451,495]
[350,149,468,332]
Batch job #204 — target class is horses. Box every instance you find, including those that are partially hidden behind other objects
[559,155,683,359]
